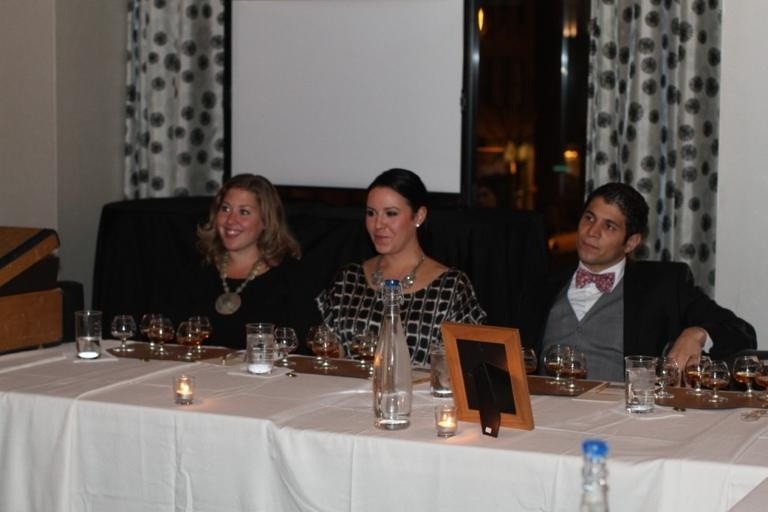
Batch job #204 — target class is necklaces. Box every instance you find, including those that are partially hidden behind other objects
[215,251,262,314]
[372,251,425,288]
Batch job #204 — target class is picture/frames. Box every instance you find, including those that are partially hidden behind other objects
[442,323,536,436]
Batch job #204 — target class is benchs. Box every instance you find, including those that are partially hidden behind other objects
[89,184,544,357]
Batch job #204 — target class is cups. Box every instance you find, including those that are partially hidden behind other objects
[247,323,274,374]
[75,310,102,359]
[624,355,657,413]
[172,372,194,404]
[430,342,454,397]
[434,405,457,437]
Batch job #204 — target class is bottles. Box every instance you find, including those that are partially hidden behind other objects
[580,441,607,512]
[374,279,412,430]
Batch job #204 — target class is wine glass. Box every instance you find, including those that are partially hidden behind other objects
[363,338,381,379]
[701,361,730,402]
[312,332,337,369]
[177,321,203,360]
[140,314,164,353]
[149,319,174,358]
[522,348,537,373]
[652,358,680,399]
[305,325,331,365]
[187,315,212,354]
[685,355,712,396]
[111,315,137,352]
[352,333,375,369]
[731,356,760,398]
[543,344,571,385]
[755,359,768,400]
[565,350,586,388]
[274,327,299,367]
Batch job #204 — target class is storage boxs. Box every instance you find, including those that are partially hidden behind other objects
[0,225,86,351]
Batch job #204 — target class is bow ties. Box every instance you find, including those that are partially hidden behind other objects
[573,268,616,297]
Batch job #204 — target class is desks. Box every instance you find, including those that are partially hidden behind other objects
[0,340,767,511]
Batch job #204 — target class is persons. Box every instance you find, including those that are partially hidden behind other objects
[158,172,322,357]
[519,183,757,382]
[315,168,486,366]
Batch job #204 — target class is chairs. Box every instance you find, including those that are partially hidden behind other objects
[638,259,696,287]
